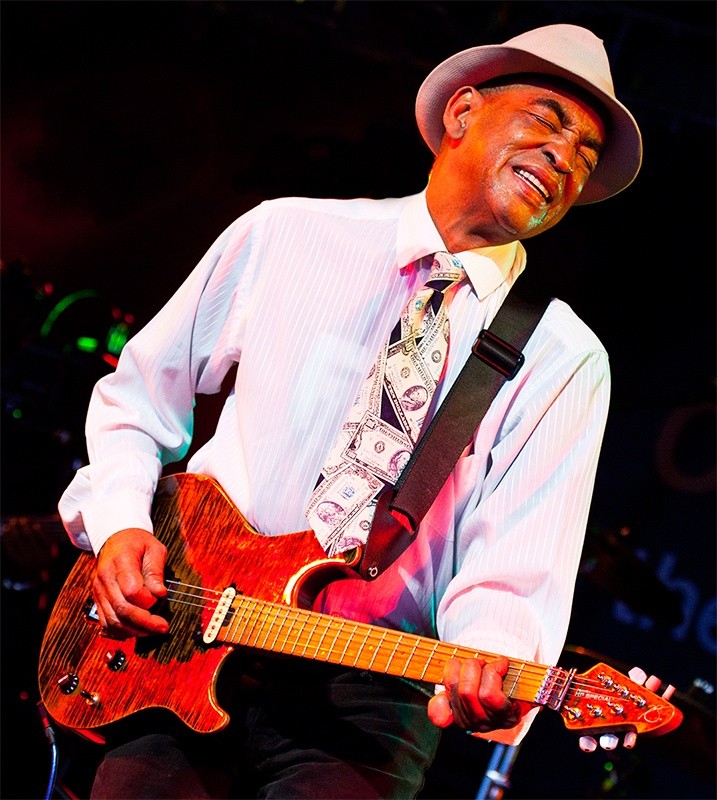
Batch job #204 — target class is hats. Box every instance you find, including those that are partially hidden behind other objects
[415,24,643,206]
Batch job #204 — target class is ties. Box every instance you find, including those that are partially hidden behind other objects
[302,248,468,562]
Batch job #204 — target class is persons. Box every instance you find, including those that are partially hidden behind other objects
[59,24,643,800]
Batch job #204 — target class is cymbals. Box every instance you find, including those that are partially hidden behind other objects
[565,644,716,720]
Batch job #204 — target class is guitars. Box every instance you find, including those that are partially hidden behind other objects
[38,472,683,753]
